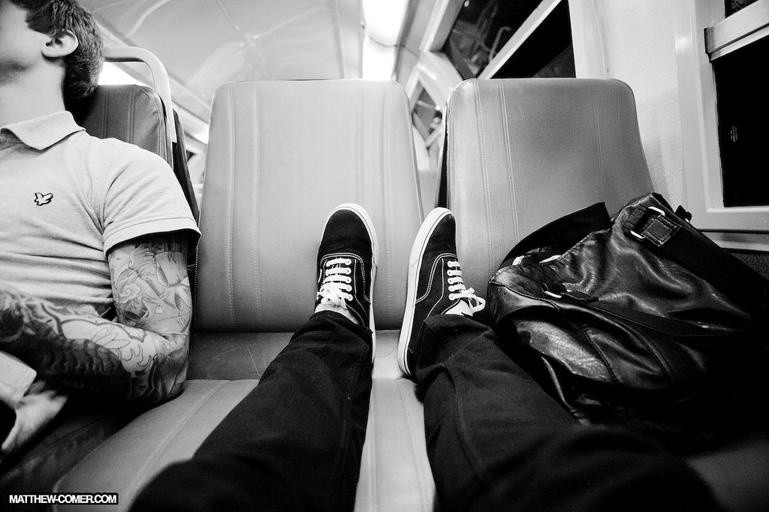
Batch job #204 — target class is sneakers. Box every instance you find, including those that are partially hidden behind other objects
[314,204,378,367]
[397,207,484,375]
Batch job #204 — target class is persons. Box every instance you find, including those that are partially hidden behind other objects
[133,202,708,506]
[0,0,201,468]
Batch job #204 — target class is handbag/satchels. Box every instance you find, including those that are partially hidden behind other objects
[486,192,769,451]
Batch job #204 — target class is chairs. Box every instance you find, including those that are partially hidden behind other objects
[0,46,176,511]
[448,78,768,510]
[55,79,442,511]
